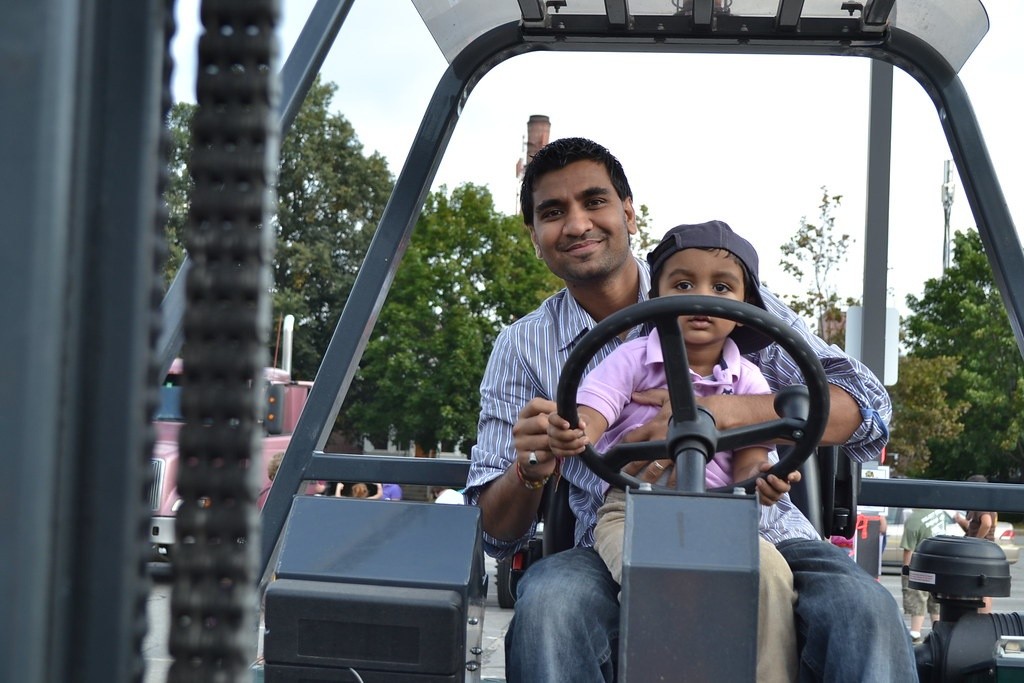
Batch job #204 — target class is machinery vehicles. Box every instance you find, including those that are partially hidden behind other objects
[143,313,329,583]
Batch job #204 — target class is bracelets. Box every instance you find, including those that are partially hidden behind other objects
[516,457,550,490]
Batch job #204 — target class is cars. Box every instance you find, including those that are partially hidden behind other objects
[856,473,1021,576]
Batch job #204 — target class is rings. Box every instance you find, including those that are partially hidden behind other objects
[529,450,538,465]
[654,461,665,470]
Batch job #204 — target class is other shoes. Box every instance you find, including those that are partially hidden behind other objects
[909,634,922,645]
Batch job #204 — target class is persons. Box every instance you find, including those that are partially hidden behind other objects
[316,482,383,499]
[463,137,919,683]
[259,451,284,510]
[431,486,464,505]
[954,475,997,614]
[547,220,801,683]
[900,509,947,643]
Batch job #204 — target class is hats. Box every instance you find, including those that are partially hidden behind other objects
[645,219,777,356]
[966,474,987,483]
